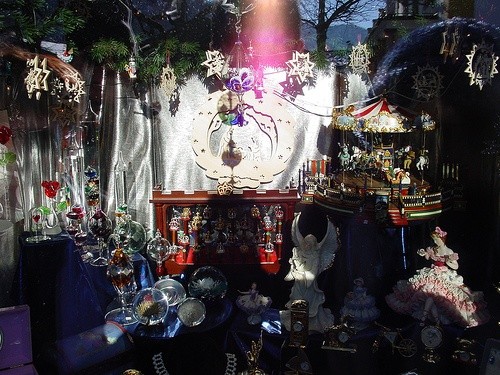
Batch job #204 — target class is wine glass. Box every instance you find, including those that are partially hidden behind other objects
[26,189,142,325]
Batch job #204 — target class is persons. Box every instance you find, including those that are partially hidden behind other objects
[340,277,380,322]
[163,204,290,265]
[236,281,272,325]
[384,227,493,329]
[279,212,338,334]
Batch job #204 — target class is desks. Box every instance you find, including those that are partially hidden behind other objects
[109,287,482,374]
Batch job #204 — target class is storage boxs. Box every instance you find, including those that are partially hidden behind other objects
[0,305,42,375]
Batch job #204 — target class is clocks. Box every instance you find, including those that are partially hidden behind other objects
[338,332,349,343]
[461,354,468,362]
[421,326,442,348]
[293,321,303,332]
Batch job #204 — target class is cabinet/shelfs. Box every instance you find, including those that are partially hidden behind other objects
[22,218,160,364]
[152,192,300,277]
